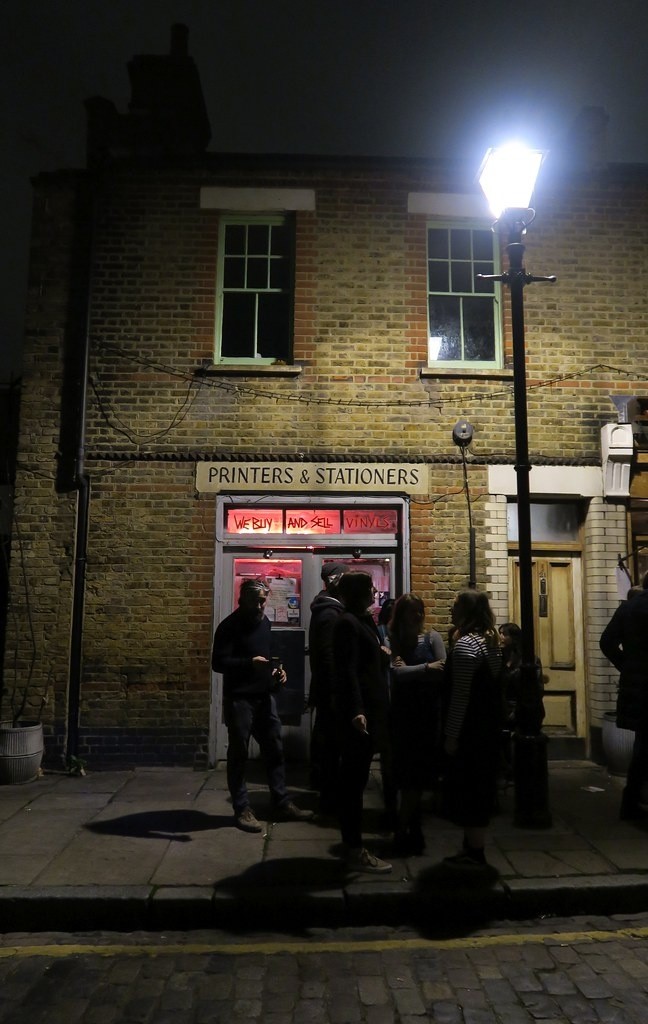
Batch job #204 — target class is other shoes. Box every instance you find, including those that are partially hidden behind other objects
[271,800,313,821]
[343,848,392,873]
[234,805,263,831]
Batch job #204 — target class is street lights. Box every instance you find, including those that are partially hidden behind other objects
[476,138,555,831]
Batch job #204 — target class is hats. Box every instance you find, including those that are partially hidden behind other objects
[320,562,350,590]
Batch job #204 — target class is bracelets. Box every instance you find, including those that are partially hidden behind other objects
[424,662,429,670]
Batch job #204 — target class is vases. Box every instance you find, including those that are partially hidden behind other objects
[0,720,44,785]
[602,712,636,775]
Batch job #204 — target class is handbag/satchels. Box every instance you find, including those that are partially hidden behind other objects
[425,634,451,711]
[464,633,504,738]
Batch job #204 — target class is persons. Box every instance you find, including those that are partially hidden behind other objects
[322,571,392,874]
[498,623,544,785]
[599,569,648,820]
[376,599,395,645]
[436,591,504,873]
[309,563,351,823]
[211,580,314,831]
[384,594,447,855]
